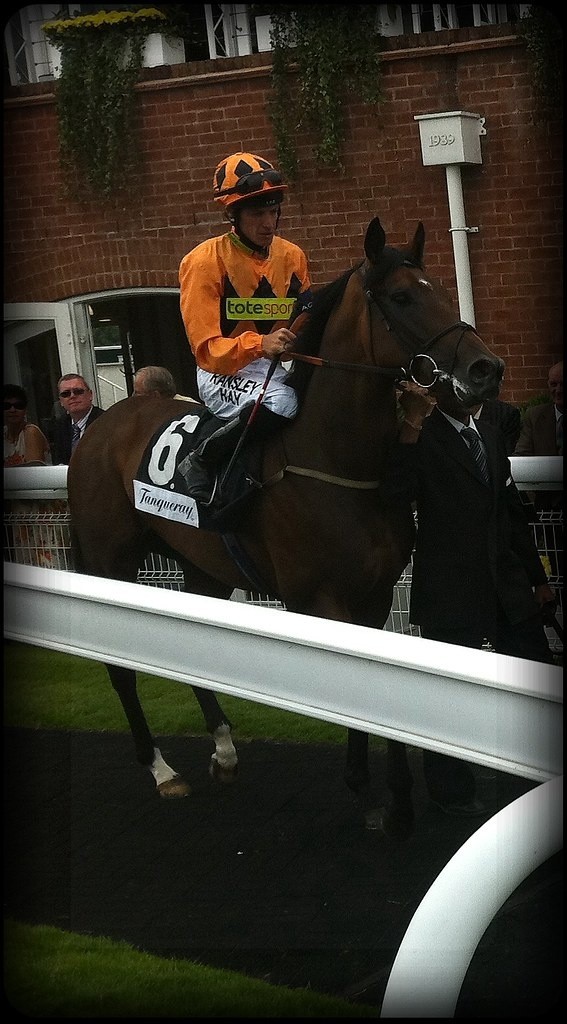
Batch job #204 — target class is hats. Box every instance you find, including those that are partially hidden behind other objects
[213,152,289,207]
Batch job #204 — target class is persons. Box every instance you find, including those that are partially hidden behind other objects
[473,398,521,457]
[130,366,177,400]
[48,373,106,507]
[173,152,314,503]
[389,383,558,818]
[511,361,563,511]
[1,382,65,566]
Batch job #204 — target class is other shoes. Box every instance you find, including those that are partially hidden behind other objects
[426,791,489,819]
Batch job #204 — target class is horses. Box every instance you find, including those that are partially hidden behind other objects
[66,216,504,798]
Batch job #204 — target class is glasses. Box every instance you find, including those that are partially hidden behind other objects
[60,388,87,398]
[3,402,26,410]
[213,169,283,199]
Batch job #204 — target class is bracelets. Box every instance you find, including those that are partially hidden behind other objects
[404,418,422,430]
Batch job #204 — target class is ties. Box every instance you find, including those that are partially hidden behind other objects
[71,425,80,455]
[463,427,491,483]
[555,415,564,456]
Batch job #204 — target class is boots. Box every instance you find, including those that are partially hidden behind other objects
[177,415,248,506]
